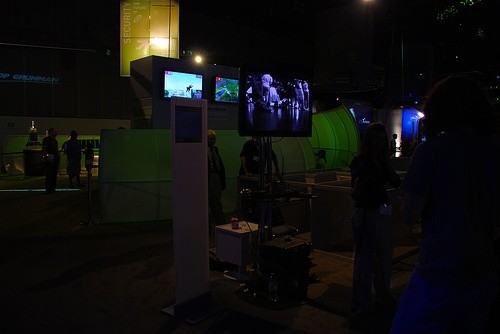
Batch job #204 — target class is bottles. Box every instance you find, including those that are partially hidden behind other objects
[231,216,238,229]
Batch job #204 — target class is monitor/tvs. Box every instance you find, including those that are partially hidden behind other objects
[235,73,312,138]
[161,69,205,99]
[213,75,240,105]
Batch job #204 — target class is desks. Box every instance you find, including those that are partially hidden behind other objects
[214,222,268,275]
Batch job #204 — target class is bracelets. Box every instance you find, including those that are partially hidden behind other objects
[244,168,247,170]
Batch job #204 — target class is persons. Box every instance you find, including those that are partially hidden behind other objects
[239,135,280,220]
[207,130,227,229]
[390,134,397,157]
[350,123,402,315]
[389,71,500,334]
[42,128,61,193]
[83,143,94,173]
[58,130,82,186]
[246,73,309,111]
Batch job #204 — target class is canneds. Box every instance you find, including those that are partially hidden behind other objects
[232,217,239,229]
